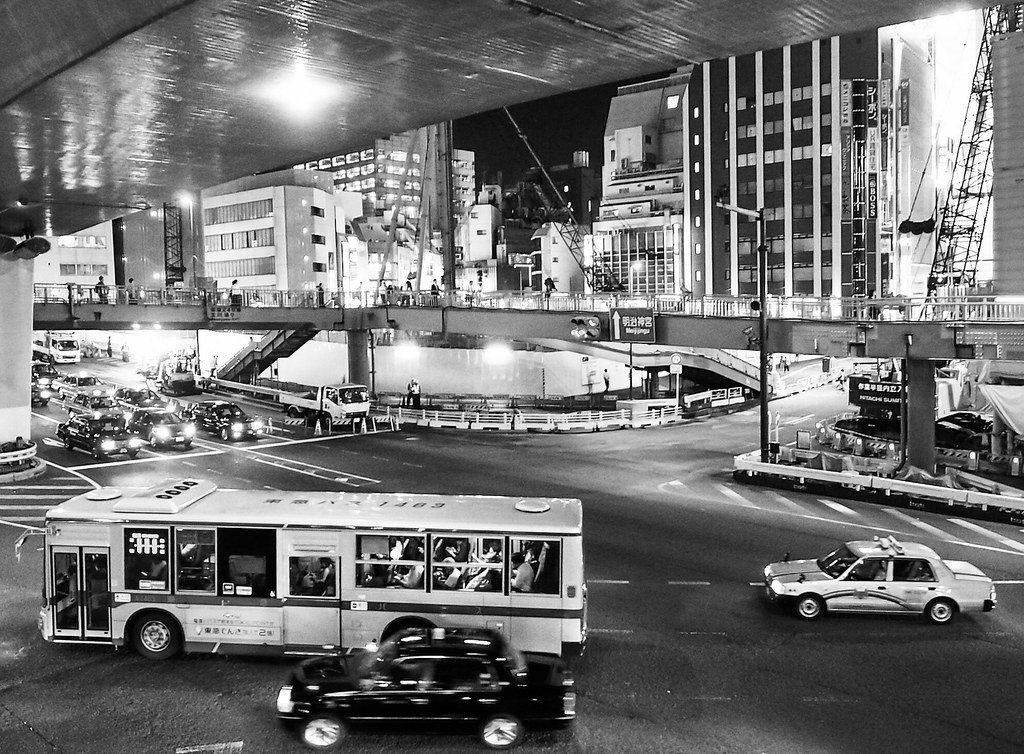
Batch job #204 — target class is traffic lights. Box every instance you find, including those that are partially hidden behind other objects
[570,329,599,340]
[569,317,599,329]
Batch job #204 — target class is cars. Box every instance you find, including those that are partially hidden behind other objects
[32,378,51,407]
[763,534,998,625]
[275,624,577,752]
[32,363,63,390]
[50,372,113,409]
[114,387,168,413]
[56,412,142,461]
[187,399,261,442]
[934,412,1023,461]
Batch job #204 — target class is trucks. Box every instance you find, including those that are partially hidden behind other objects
[279,382,372,430]
[32,336,81,367]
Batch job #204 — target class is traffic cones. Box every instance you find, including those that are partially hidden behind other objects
[310,419,323,437]
[393,415,401,432]
[266,417,276,435]
[359,417,369,434]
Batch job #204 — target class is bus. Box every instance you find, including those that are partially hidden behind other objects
[17,479,588,667]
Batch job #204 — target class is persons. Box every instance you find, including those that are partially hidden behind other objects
[542,276,558,310]
[248,335,255,342]
[143,351,220,380]
[836,363,891,393]
[125,277,174,304]
[65,565,81,612]
[304,282,325,309]
[147,535,197,580]
[603,368,609,394]
[300,558,334,594]
[95,275,108,302]
[364,535,545,593]
[380,280,413,307]
[352,281,363,308]
[776,355,789,372]
[407,378,421,408]
[465,281,477,307]
[430,279,442,305]
[227,280,241,312]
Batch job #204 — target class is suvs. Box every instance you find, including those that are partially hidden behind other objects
[127,407,196,449]
